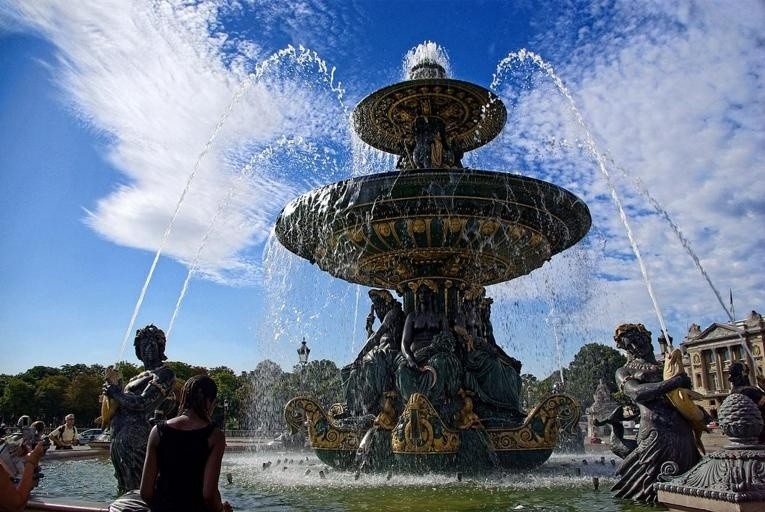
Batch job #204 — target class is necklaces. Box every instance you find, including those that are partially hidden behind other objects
[127,363,167,381]
[621,362,662,370]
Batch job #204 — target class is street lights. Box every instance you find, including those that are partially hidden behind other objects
[297,337,310,396]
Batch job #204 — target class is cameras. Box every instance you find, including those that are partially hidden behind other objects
[32,437,51,454]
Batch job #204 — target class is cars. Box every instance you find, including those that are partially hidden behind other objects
[77,429,103,446]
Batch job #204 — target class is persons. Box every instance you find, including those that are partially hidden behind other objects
[47,413,81,451]
[393,276,463,413]
[446,281,523,418]
[721,357,765,414]
[337,287,406,421]
[0,410,51,511]
[612,321,711,503]
[99,322,177,501]
[138,373,234,511]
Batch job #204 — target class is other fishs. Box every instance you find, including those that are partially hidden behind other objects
[93,364,123,432]
[663,348,707,456]
